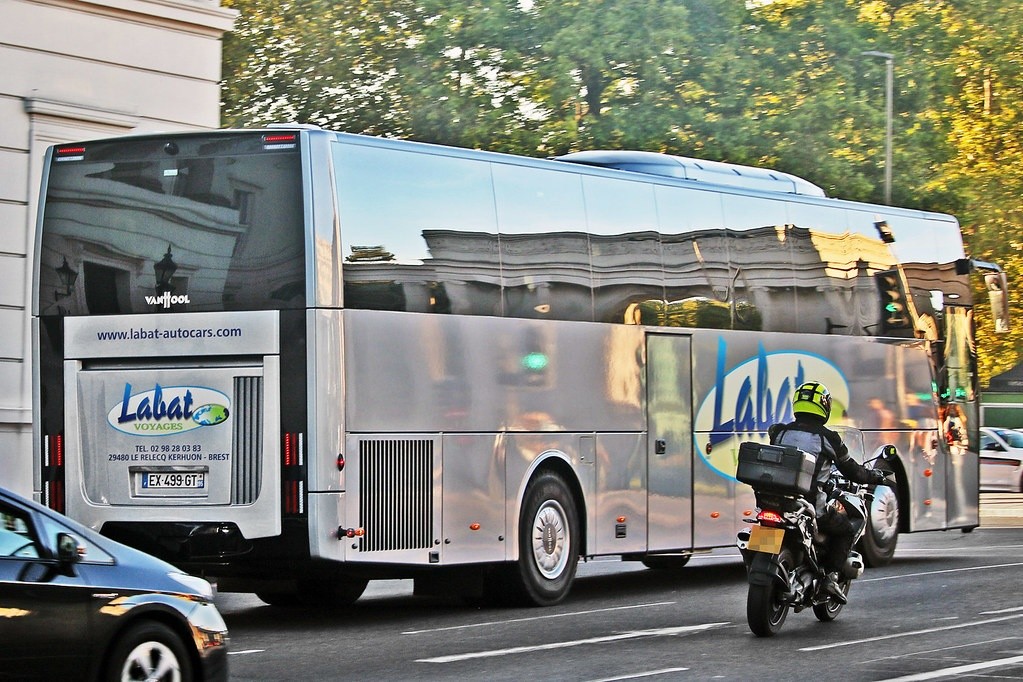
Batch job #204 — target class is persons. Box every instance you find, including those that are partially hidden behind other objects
[768,381,884,605]
[948,422,962,445]
[984,435,1008,452]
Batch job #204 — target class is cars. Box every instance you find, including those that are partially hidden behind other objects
[0,488,231,682]
[976,426,1023,493]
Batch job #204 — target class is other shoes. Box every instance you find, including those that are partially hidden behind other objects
[819,571,847,604]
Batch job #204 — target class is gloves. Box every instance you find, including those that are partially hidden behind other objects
[870,469,887,484]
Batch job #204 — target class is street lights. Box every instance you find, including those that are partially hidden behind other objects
[860,49,894,205]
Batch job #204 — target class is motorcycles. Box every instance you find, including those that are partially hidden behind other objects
[735,423,897,637]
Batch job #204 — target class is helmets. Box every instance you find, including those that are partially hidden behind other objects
[791,381,832,425]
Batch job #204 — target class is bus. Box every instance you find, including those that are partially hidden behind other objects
[29,118,1011,613]
